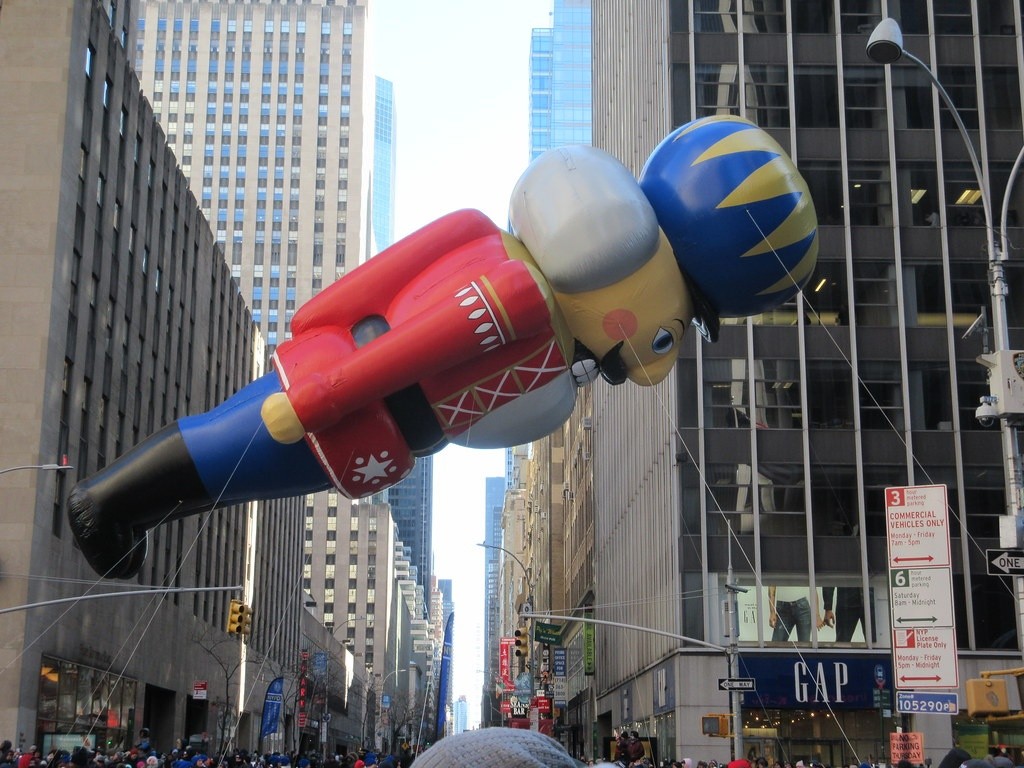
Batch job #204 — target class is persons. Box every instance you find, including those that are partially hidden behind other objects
[0,728,431,768]
[768,585,876,643]
[67,115,818,580]
[580,731,850,768]
[938,748,1015,768]
[408,727,578,768]
[858,760,924,768]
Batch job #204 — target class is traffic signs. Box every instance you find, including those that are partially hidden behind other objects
[718,677,756,692]
[986,548,1024,576]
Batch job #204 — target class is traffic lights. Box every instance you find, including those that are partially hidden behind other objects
[514,627,529,658]
[242,604,253,635]
[227,600,244,635]
[702,716,728,735]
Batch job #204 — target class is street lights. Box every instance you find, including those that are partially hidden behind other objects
[476,670,503,727]
[476,543,535,705]
[378,669,406,750]
[865,17,1024,630]
[323,617,365,761]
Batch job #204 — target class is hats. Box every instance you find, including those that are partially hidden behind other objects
[353,752,395,768]
[860,762,871,768]
[796,760,804,766]
[407,727,577,768]
[30,728,311,768]
[620,731,629,739]
